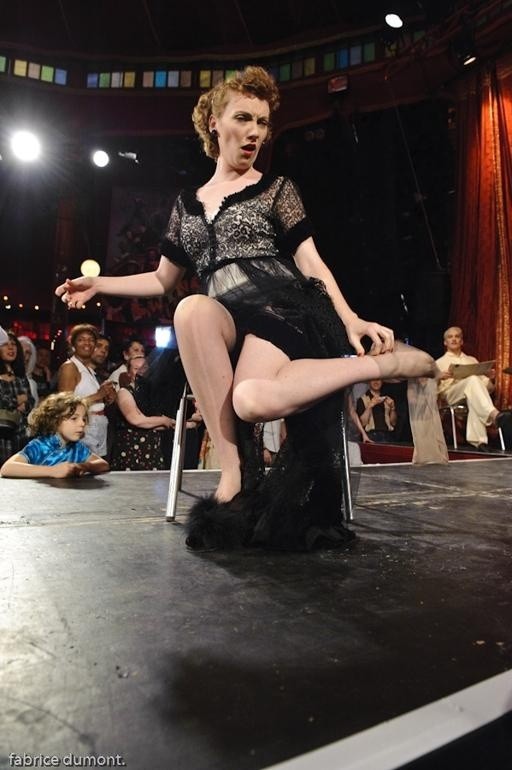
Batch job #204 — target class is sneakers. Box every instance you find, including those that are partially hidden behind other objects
[492,410,512,428]
[477,442,493,454]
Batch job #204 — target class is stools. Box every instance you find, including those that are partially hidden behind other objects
[440,405,507,451]
[164,380,356,525]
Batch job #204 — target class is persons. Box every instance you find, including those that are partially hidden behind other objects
[54,67,440,550]
